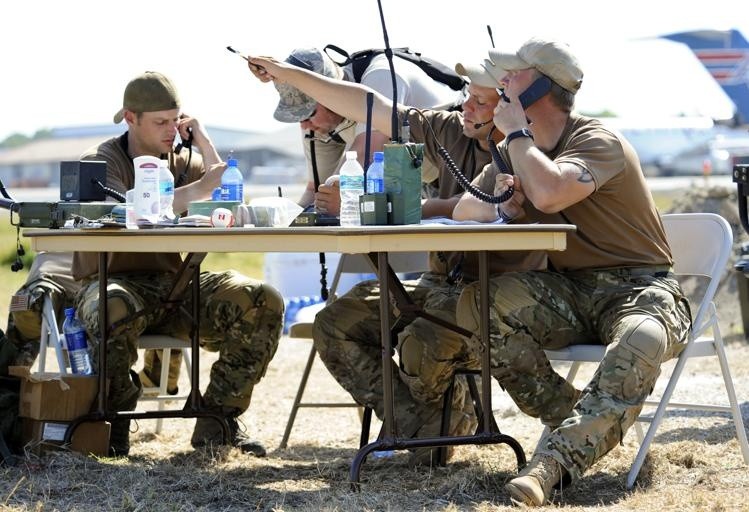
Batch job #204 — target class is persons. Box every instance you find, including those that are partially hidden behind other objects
[243,54,552,471]
[453,36,693,508]
[272,41,469,209]
[70,70,288,460]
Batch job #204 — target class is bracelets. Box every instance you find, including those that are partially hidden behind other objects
[505,129,535,148]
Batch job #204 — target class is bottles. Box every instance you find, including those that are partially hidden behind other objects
[340,151,365,228]
[159,159,175,221]
[64,310,91,374]
[219,160,243,201]
[283,295,323,333]
[367,152,384,194]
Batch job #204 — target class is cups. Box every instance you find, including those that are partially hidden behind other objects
[233,205,244,227]
[247,206,276,228]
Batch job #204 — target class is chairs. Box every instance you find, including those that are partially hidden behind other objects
[518,205,749,494]
[276,233,441,454]
[31,248,202,439]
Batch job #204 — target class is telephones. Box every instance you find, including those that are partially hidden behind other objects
[177,115,193,148]
[518,77,551,111]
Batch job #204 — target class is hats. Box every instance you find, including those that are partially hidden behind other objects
[113,70,181,125]
[455,56,505,90]
[272,47,347,124]
[488,38,584,96]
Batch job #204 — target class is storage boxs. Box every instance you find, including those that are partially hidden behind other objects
[6,362,112,423]
[9,411,113,466]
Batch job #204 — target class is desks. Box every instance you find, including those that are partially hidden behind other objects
[16,216,580,495]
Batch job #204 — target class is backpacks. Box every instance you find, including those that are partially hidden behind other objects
[323,43,470,92]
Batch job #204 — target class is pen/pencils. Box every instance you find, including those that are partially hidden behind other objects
[278,186,282,197]
[227,46,267,74]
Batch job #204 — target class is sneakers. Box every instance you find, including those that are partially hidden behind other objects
[109,406,136,458]
[454,391,479,437]
[504,453,567,508]
[190,411,268,458]
[407,409,470,474]
